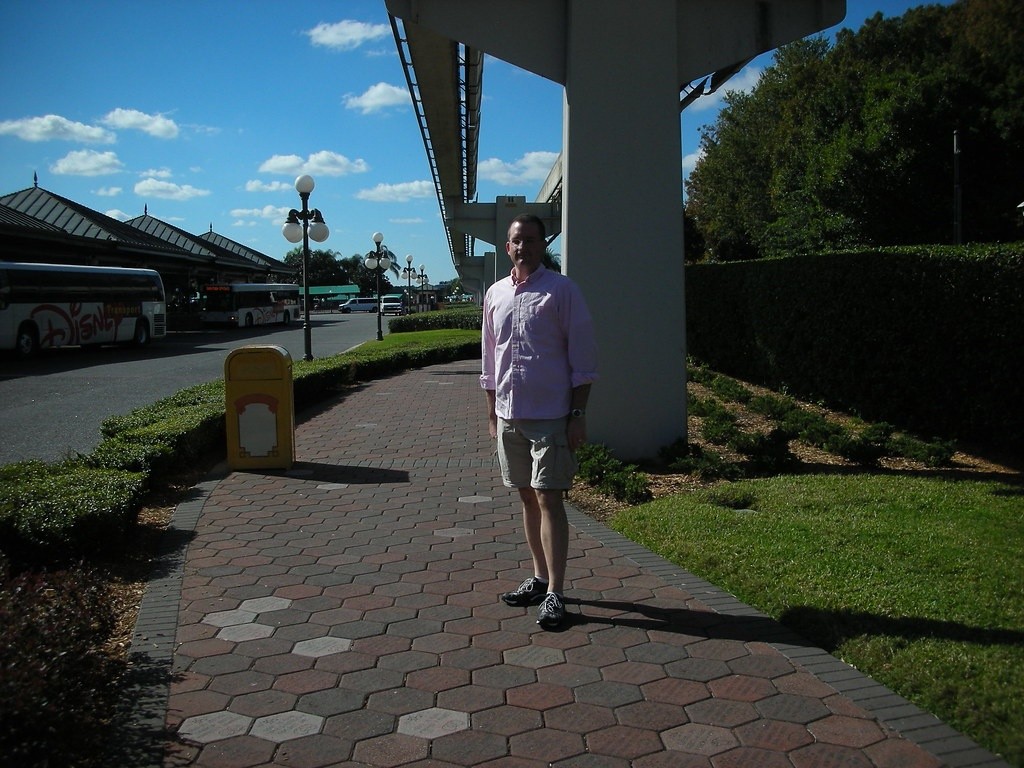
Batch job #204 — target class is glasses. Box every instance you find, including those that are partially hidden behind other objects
[508,238,527,247]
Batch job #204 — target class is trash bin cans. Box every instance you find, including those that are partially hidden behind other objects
[225,344,295,470]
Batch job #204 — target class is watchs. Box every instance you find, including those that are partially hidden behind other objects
[571,409,585,417]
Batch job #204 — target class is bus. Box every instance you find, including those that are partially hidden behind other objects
[0,260,167,364]
[198,283,301,331]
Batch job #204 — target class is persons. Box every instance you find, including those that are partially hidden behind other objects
[480,212,599,627]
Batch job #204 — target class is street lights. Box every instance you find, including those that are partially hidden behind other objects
[401,255,417,316]
[363,232,391,341]
[415,264,429,313]
[282,174,329,362]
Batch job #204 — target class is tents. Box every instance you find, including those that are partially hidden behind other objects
[327,294,349,309]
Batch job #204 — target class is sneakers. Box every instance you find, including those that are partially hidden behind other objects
[535,592,566,626]
[502,576,549,605]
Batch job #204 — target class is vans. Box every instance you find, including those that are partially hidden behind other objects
[380,294,403,316]
[338,298,377,314]
